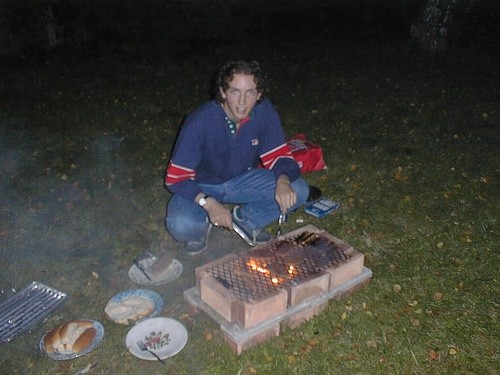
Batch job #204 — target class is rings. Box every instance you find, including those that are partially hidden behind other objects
[214,221,219,228]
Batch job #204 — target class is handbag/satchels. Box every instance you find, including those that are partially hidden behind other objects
[286,133,325,172]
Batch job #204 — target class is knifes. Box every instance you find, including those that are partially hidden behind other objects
[232,221,255,246]
[133,259,152,281]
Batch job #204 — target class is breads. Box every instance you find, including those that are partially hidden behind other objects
[43,320,97,355]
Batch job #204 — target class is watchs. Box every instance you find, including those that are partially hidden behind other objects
[197,194,211,207]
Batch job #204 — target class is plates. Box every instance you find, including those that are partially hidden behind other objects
[43,319,104,360]
[128,256,183,286]
[125,317,188,360]
[104,288,165,326]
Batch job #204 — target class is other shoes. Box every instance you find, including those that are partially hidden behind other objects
[186,222,212,255]
[229,206,269,245]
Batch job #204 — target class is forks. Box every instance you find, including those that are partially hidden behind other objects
[137,340,166,365]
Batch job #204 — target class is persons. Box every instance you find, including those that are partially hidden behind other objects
[163,53,309,257]
